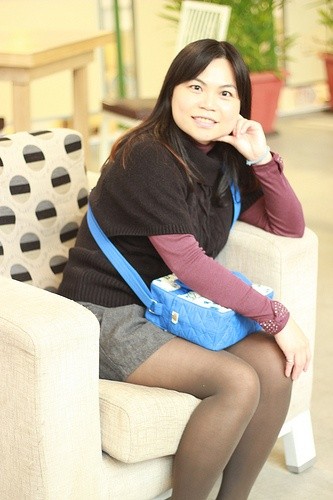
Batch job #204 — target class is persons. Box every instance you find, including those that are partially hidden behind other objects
[59,37,314,500]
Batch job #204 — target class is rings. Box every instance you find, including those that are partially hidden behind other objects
[286,360,294,362]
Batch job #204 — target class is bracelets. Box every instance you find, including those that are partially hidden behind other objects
[246,147,271,167]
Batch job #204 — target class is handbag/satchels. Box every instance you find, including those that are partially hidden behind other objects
[144,267,274,351]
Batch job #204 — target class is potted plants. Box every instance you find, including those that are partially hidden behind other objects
[157,0,296,136]
[316,0,333,110]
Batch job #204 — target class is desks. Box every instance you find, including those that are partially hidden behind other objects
[0,28,116,171]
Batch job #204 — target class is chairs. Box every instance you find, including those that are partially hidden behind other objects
[0,128,317,500]
[98,0,232,171]
